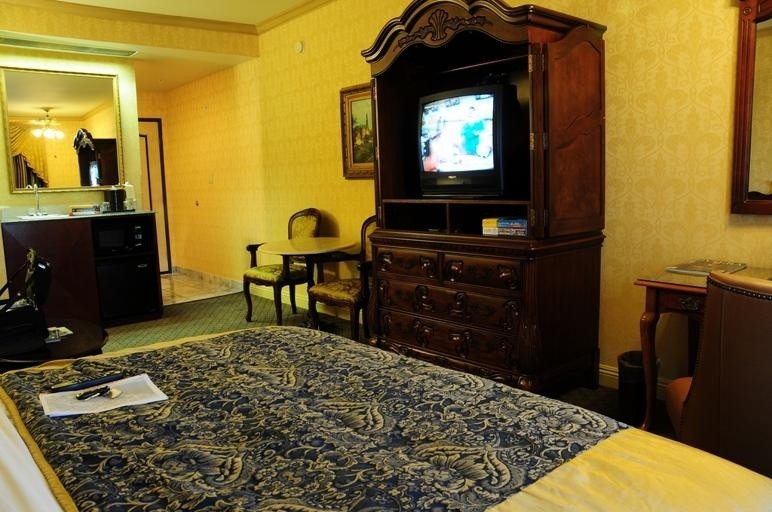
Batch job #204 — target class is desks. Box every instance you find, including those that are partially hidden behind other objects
[633,261,772,432]
[274,238,355,330]
[0,320,109,366]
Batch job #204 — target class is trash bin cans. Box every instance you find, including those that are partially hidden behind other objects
[617,352,643,427]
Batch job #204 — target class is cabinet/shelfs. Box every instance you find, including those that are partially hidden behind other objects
[362,0,608,396]
[4,211,165,326]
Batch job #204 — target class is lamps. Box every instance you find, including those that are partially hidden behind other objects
[30,107,65,139]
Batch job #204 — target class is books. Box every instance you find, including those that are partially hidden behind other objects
[44,329,61,343]
[482,228,528,237]
[664,258,747,276]
[481,217,527,228]
[68,204,103,216]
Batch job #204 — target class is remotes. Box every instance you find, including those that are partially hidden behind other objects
[50,369,126,392]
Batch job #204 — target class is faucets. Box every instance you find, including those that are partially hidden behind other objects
[26,184,32,189]
[34,183,40,215]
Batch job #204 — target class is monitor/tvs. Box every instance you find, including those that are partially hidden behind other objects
[86,162,98,185]
[415,82,524,200]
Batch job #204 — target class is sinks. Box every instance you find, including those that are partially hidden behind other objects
[17,213,69,221]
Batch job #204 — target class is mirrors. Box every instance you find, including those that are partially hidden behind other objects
[0,66,126,194]
[730,0,772,215]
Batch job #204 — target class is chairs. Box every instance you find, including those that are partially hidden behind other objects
[664,271,772,479]
[308,215,378,342]
[243,208,321,326]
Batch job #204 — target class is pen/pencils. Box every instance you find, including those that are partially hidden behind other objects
[76,385,110,401]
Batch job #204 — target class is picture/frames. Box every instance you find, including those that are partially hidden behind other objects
[339,82,377,179]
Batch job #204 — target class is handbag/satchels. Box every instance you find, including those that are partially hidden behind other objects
[0,248,52,358]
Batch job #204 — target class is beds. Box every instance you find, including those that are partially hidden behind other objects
[0,325,772,512]
